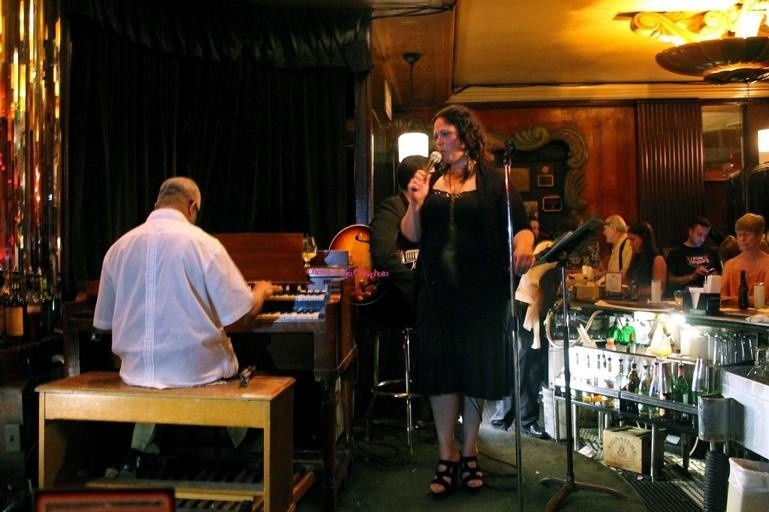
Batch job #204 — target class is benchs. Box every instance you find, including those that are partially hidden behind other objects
[32,368,299,512]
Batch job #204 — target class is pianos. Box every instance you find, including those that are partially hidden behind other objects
[61,231,355,512]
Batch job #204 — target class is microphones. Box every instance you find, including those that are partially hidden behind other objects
[412,150,443,191]
[503,143,516,163]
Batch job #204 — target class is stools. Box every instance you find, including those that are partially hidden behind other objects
[361,323,430,457]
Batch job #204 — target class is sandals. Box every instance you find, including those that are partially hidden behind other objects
[429,459,458,499]
[460,456,484,494]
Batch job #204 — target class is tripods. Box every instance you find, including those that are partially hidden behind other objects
[539,256,626,512]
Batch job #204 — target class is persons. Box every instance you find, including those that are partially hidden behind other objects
[402,103,536,498]
[93,174,277,482]
[517,206,769,308]
[368,153,445,325]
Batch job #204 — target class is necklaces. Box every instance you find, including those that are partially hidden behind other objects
[448,167,472,201]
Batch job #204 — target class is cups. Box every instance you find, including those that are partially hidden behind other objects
[692,331,769,379]
[651,279,662,303]
[672,289,685,308]
[593,331,607,348]
[688,287,705,309]
[753,281,766,308]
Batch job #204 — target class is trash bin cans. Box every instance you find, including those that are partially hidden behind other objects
[726,458,769,512]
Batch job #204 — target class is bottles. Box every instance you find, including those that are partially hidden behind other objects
[1,266,64,344]
[606,317,636,349]
[659,324,680,355]
[555,352,705,428]
[629,269,639,302]
[738,270,749,310]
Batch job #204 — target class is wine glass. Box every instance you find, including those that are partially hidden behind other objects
[302,237,318,269]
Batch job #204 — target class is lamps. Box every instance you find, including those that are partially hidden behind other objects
[628,2,769,87]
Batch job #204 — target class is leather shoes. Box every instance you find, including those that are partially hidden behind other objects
[521,423,548,438]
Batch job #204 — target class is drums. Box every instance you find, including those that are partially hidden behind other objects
[328,224,386,305]
